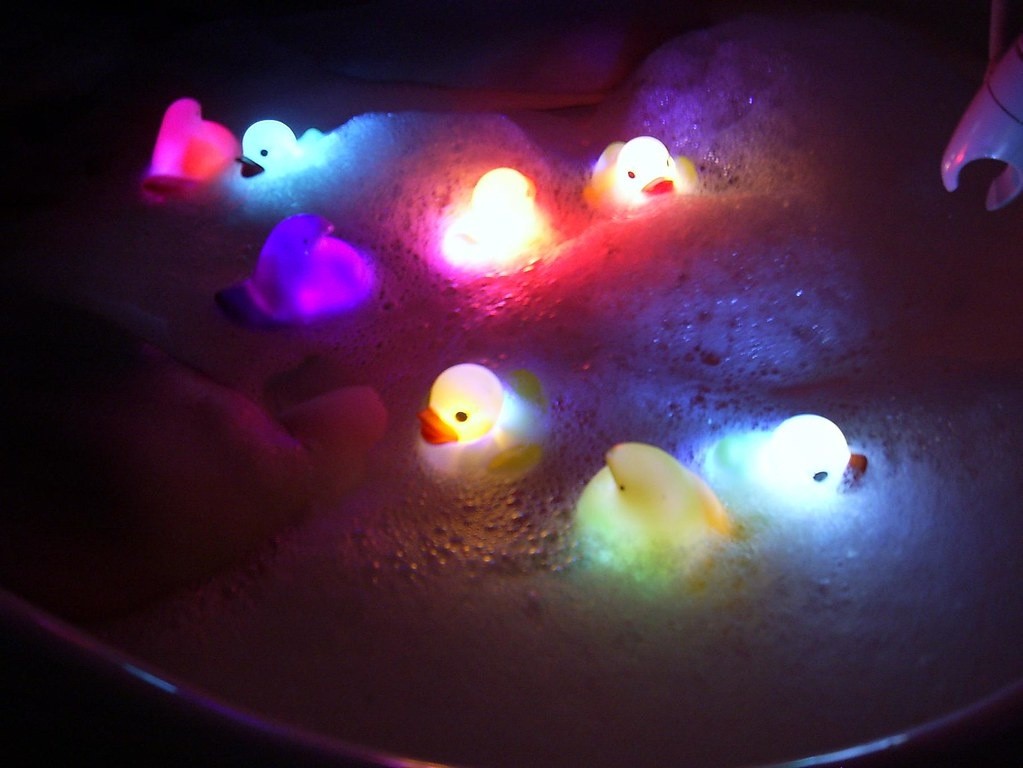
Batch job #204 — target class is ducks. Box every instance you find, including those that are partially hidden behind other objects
[580,134,700,215]
[249,212,382,324]
[572,438,732,591]
[411,361,556,499]
[704,412,869,511]
[438,166,551,273]
[149,95,242,182]
[235,116,339,180]
